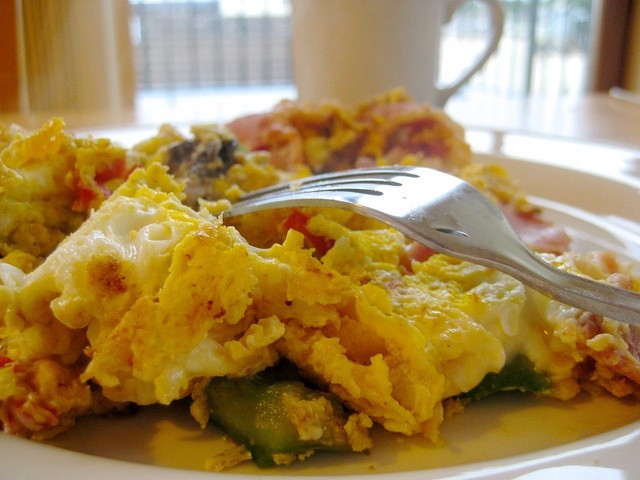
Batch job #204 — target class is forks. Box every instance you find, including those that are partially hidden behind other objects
[223,166,640,327]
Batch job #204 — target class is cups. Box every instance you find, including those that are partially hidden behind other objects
[292,0,505,111]
